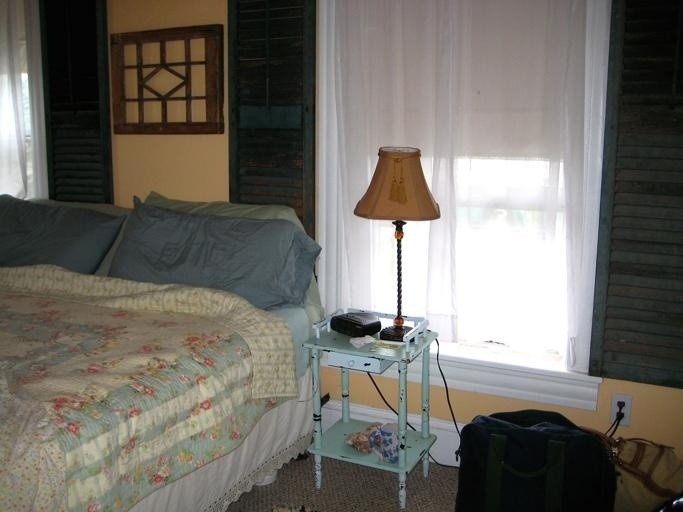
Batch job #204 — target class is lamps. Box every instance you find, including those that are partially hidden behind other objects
[352,145,442,342]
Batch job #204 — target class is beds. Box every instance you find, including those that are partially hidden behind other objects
[0,193,324,512]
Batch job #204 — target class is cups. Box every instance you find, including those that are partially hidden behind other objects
[381,421,399,464]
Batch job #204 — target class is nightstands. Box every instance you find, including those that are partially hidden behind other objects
[298,330,440,509]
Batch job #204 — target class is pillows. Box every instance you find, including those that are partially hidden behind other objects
[1,194,133,281]
[108,190,324,312]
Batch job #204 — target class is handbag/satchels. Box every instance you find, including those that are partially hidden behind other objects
[614,436,683,511]
[453,409,618,511]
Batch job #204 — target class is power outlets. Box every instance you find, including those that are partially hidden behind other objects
[611,392,633,427]
